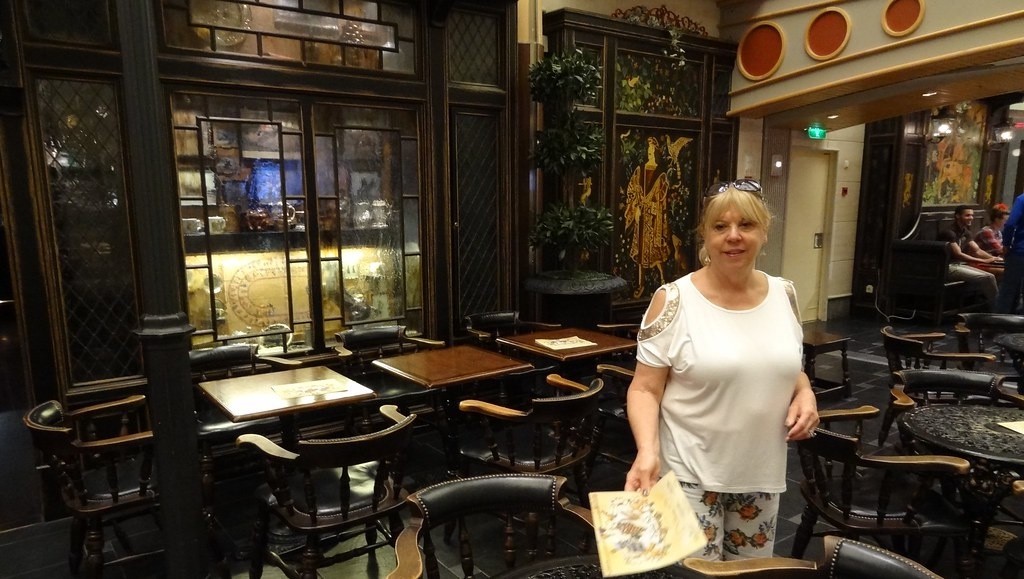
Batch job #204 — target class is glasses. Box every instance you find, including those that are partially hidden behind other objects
[702,178,767,205]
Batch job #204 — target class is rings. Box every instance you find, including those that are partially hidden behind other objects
[810,429,817,437]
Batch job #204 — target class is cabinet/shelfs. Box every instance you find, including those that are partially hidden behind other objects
[543,6,737,337]
[846,102,1009,320]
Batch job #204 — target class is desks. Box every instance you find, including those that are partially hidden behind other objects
[372,344,535,503]
[899,402,1024,558]
[198,366,377,560]
[495,329,638,431]
[992,333,1024,389]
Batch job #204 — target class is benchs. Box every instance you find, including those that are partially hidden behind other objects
[890,209,991,326]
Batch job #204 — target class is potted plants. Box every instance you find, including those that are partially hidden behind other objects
[523,45,628,324]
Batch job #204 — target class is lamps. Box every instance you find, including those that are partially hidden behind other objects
[927,105,957,145]
[987,116,1017,152]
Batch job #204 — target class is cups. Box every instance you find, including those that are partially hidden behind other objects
[209,215,226,233]
[182,218,202,235]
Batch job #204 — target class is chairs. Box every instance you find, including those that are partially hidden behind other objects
[189,311,1024,579]
[21,390,161,579]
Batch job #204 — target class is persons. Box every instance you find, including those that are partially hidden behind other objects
[974,202,1007,257]
[938,205,1004,303]
[623,178,821,570]
[997,192,1024,314]
